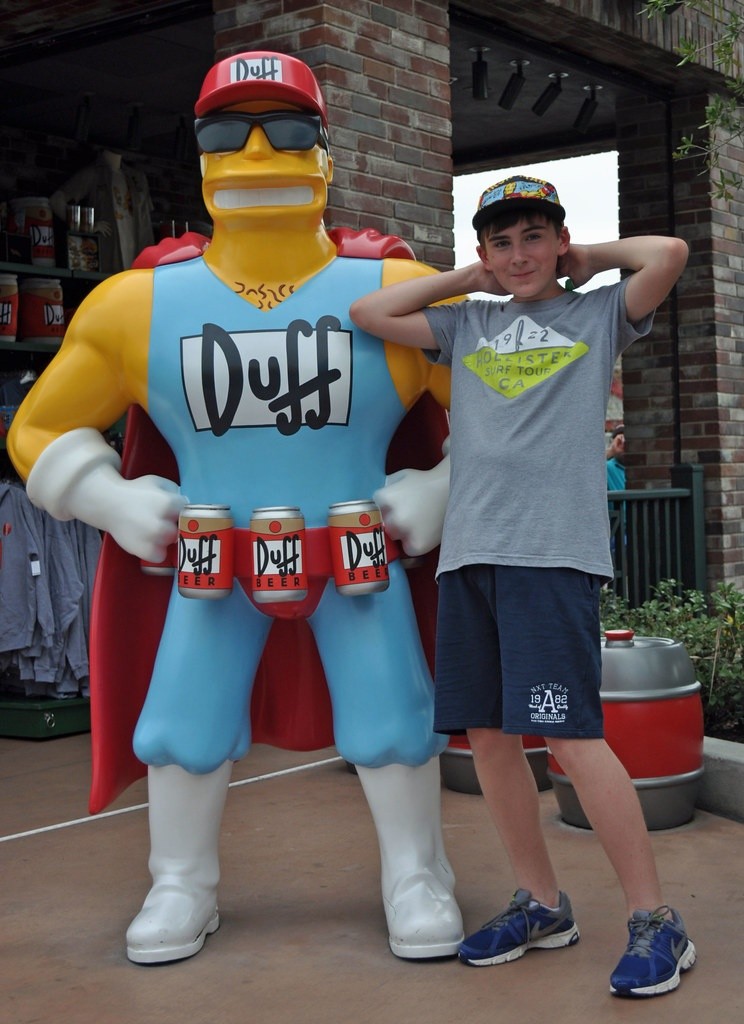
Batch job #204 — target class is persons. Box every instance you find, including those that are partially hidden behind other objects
[5,49,467,968]
[348,181,699,998]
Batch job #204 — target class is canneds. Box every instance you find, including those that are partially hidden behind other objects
[0,197,67,346]
[140,540,176,577]
[176,503,233,599]
[249,505,307,602]
[327,498,390,597]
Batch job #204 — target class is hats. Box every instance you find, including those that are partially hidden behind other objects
[472,174,566,224]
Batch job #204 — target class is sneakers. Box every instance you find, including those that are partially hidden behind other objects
[457,887,581,967]
[609,905,697,996]
